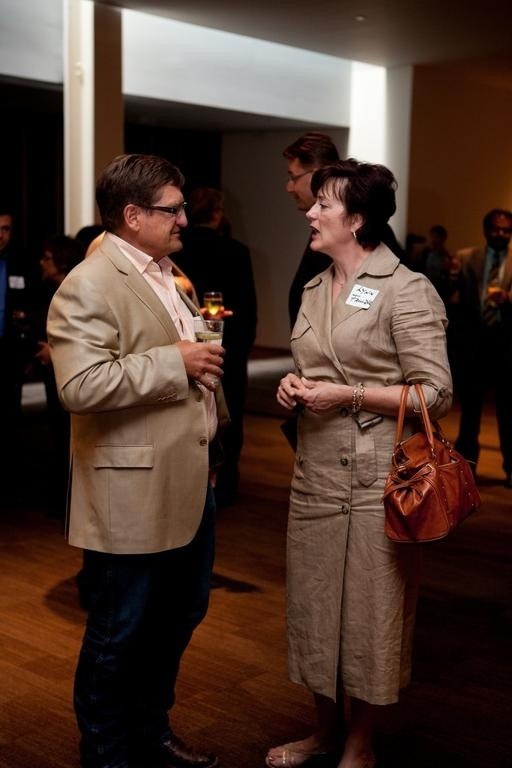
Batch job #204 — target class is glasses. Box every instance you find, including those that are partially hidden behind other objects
[135,202,187,216]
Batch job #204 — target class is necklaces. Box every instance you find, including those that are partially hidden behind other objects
[334,278,345,288]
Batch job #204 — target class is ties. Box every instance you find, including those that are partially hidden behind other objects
[484,254,500,326]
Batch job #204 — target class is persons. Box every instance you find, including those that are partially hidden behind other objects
[46,153,232,768]
[404,208,512,488]
[265,158,454,768]
[280,130,339,453]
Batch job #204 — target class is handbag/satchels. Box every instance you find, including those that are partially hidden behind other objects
[383,431,483,543]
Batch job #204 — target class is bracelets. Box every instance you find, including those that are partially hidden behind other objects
[351,383,365,415]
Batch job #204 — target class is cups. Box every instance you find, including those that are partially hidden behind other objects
[194,319,224,385]
[487,280,505,307]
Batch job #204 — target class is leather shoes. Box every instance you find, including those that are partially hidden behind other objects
[162,735,218,768]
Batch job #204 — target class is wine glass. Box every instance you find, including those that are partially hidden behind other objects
[203,293,222,320]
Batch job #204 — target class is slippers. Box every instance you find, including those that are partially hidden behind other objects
[266,743,327,768]
